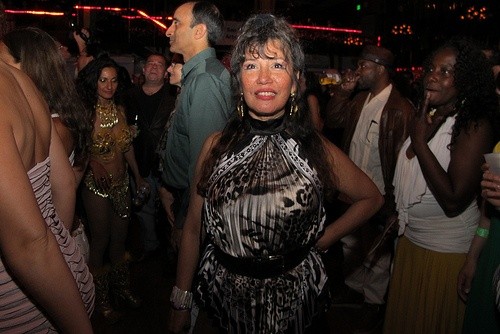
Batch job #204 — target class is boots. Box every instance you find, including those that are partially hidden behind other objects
[108,254,143,314]
[91,267,123,328]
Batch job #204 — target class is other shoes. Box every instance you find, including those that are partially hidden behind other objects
[361,303,380,326]
[330,287,362,308]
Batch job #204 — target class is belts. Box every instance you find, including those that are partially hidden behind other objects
[212,243,312,280]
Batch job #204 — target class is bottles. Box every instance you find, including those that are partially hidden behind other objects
[319,75,346,85]
[134,178,153,206]
[134,112,141,137]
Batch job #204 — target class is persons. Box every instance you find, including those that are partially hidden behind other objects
[169,13,384,334]
[0,0,500,334]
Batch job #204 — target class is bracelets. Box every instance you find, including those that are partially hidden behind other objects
[476,226,489,238]
[316,244,328,255]
[171,286,193,311]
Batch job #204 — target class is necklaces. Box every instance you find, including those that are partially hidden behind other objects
[95,102,119,128]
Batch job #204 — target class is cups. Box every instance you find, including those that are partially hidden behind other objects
[483,153,500,180]
